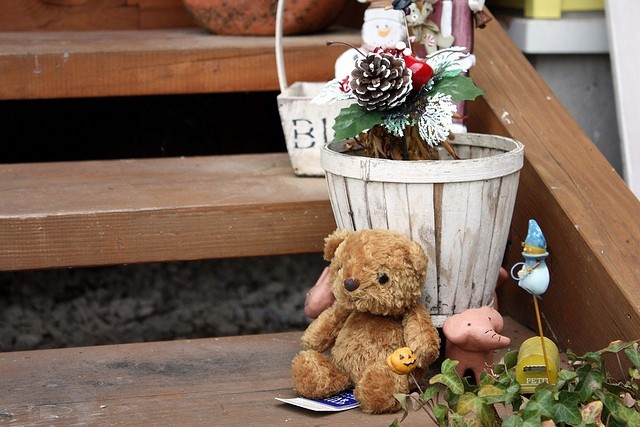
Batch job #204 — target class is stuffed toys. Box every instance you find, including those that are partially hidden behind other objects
[290,227,443,415]
[328,0,416,102]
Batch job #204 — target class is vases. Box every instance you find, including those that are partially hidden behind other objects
[276,79,355,175]
[319,132,525,328]
[320,37,487,162]
